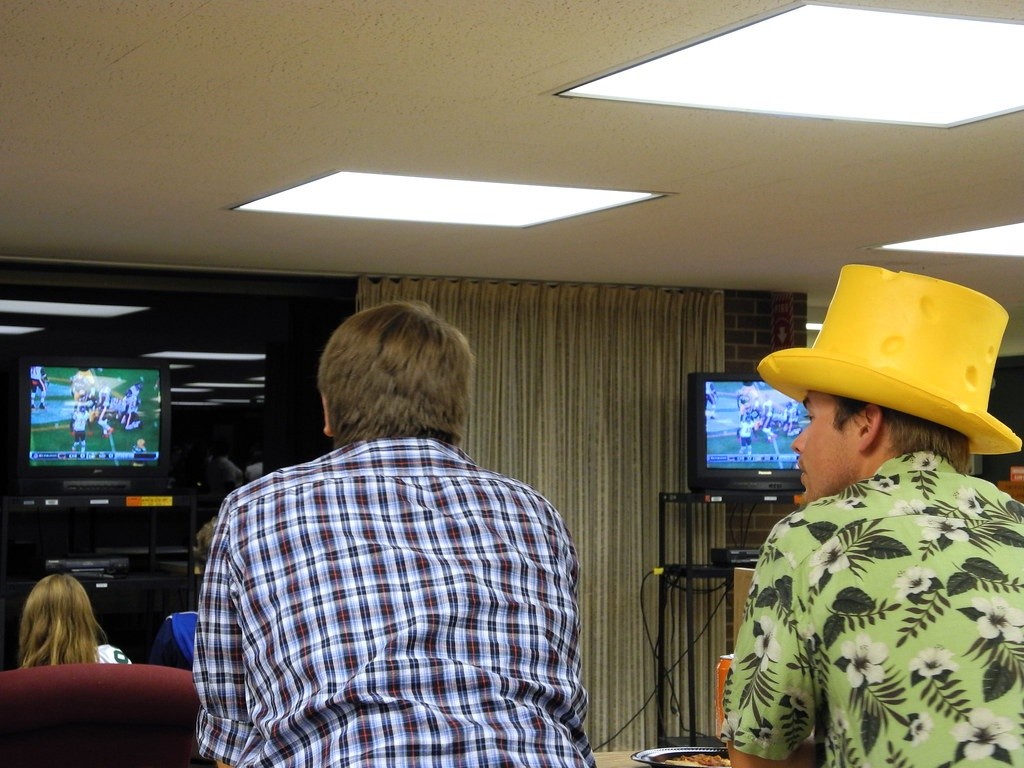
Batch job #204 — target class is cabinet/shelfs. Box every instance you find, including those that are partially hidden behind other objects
[0,492,225,663]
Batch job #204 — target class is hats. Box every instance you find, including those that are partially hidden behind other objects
[125,390,132,395]
[757,264,1022,455]
[136,383,143,390]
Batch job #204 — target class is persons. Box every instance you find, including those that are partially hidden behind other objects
[187,299,596,768]
[9,574,135,670]
[716,263,1024,768]
[705,380,803,458]
[145,513,224,667]
[197,444,266,495]
[29,366,160,464]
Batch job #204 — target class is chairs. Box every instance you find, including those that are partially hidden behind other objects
[0,664,202,768]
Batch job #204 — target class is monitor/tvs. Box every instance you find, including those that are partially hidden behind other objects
[687,373,807,493]
[18,356,171,496]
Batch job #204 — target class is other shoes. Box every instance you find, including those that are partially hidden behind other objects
[71,432,75,435]
[88,431,93,436]
[103,434,109,438]
[31,404,35,410]
[108,428,113,434]
[39,403,45,409]
